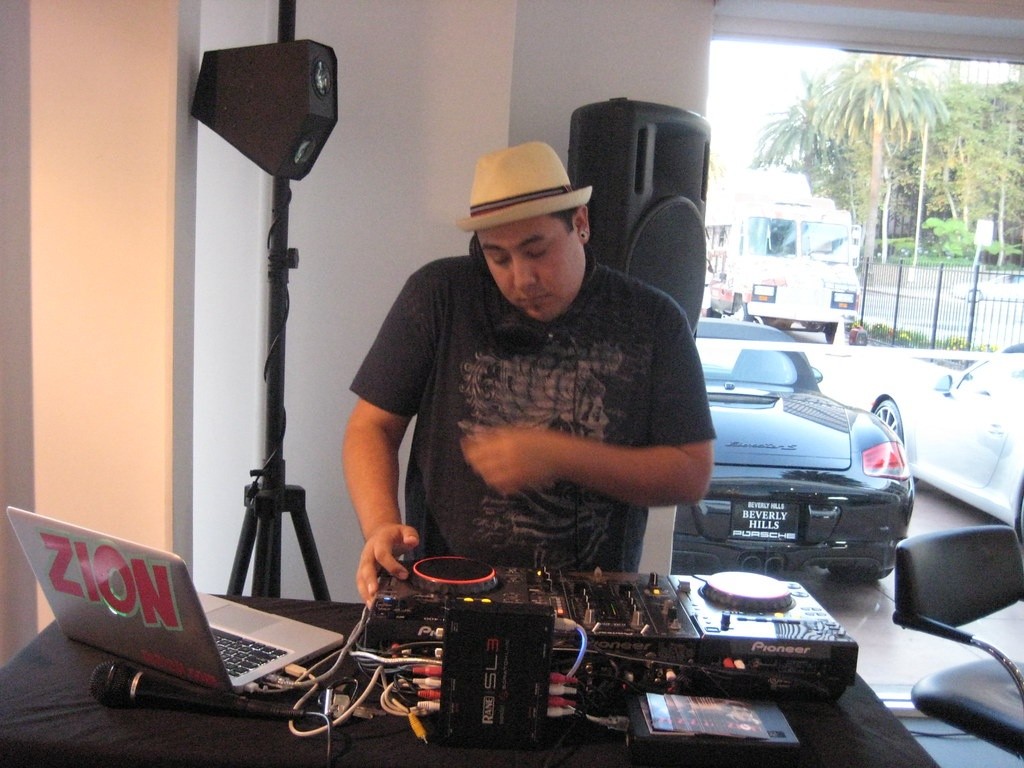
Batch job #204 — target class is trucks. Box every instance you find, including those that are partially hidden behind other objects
[704,169,864,344]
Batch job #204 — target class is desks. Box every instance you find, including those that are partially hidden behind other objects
[0,595,940,768]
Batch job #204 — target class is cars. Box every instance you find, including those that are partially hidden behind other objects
[865,344,1024,543]
[952,274,1023,304]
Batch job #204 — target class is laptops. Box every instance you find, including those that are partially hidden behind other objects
[5,505,343,693]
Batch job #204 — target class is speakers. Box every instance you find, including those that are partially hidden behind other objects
[566,96,710,341]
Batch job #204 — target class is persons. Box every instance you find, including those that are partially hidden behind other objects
[341,140,717,614]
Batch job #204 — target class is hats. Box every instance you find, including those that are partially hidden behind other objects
[452,141,594,234]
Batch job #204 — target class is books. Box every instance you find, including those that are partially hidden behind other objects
[637,691,770,740]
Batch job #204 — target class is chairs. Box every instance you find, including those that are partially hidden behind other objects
[891,525,1024,760]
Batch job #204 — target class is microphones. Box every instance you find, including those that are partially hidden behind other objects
[87,661,306,720]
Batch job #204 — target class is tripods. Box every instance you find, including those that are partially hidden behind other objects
[226,0,331,602]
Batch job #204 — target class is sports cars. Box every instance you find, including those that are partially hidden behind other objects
[673,318,915,582]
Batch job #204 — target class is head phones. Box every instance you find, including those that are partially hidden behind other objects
[467,235,595,354]
